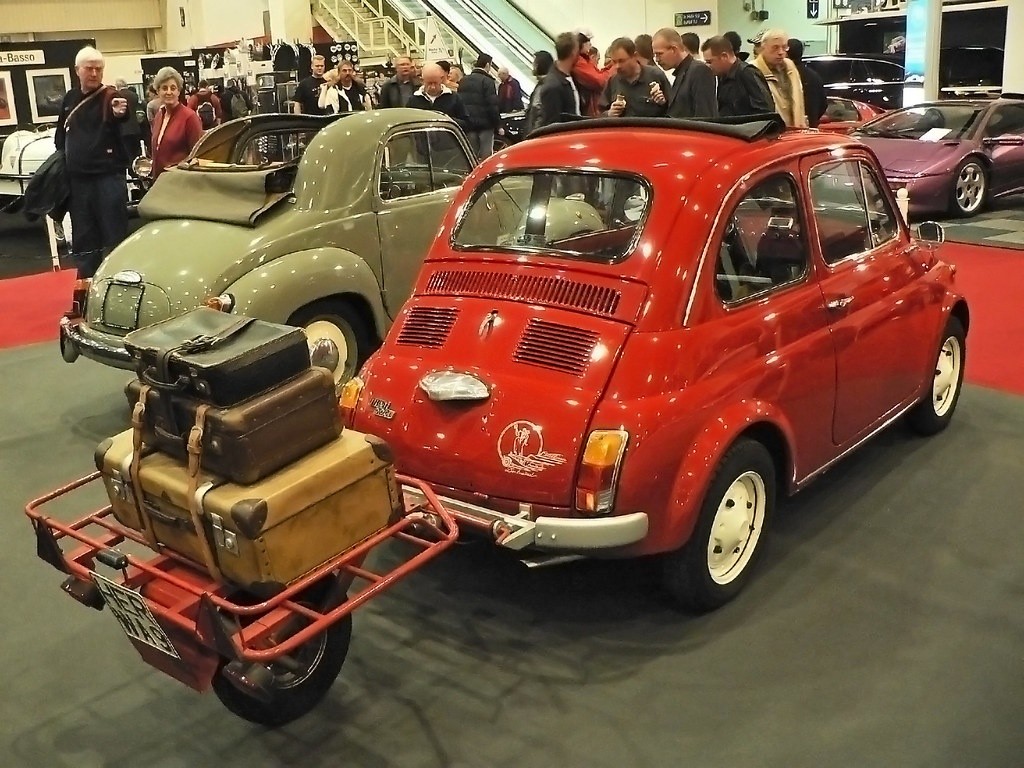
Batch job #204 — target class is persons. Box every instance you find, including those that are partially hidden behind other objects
[149,65,204,188]
[106,77,255,177]
[54,45,131,279]
[519,27,828,142]
[291,54,525,167]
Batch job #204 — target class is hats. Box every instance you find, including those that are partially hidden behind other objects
[747,28,769,44]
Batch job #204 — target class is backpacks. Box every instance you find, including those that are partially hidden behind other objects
[226,89,248,118]
[195,91,218,130]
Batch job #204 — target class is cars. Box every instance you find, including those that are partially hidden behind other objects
[330,114,968,613]
[938,47,1006,98]
[57,107,606,404]
[799,57,904,110]
[809,99,1024,219]
[0,124,153,211]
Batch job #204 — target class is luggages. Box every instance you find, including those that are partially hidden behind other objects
[94,305,405,599]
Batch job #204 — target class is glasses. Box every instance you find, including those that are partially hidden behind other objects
[771,45,789,51]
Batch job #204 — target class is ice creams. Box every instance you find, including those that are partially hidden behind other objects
[617,95,625,101]
[649,81,657,88]
